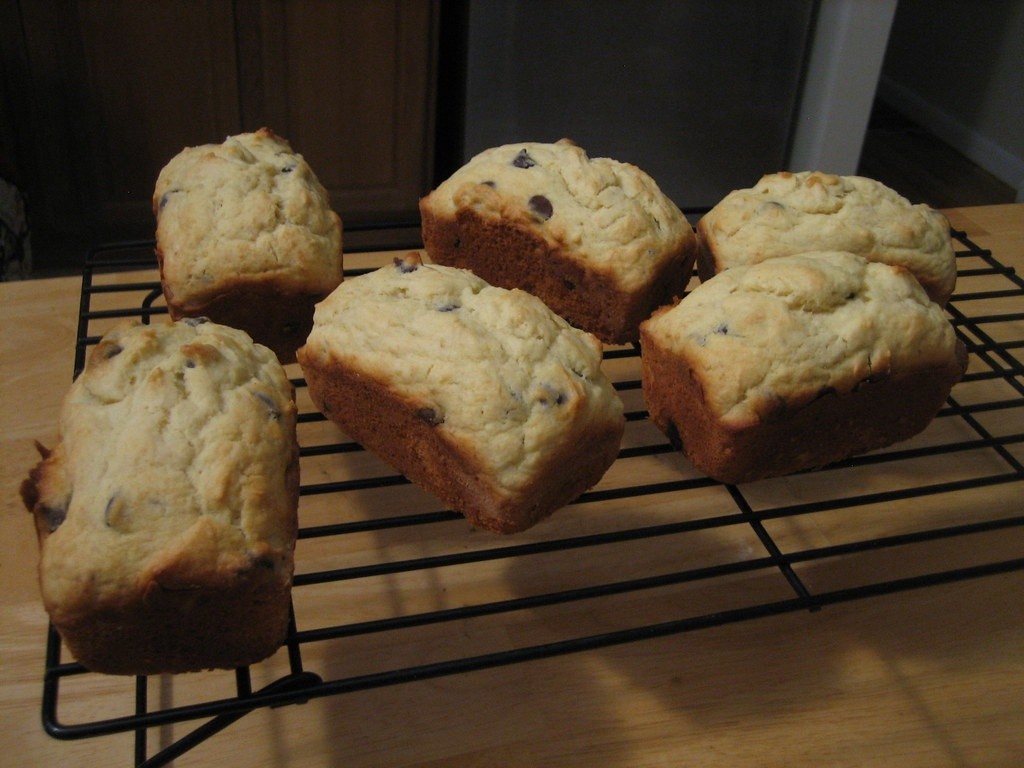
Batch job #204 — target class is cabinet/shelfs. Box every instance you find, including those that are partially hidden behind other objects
[54,0,439,245]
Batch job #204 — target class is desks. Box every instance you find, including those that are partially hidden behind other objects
[0,202,1024,768]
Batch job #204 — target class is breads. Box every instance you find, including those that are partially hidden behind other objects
[152,126,343,357]
[295,250,628,536]
[640,250,970,484]
[19,315,300,677]
[693,168,957,309]
[417,138,698,344]
